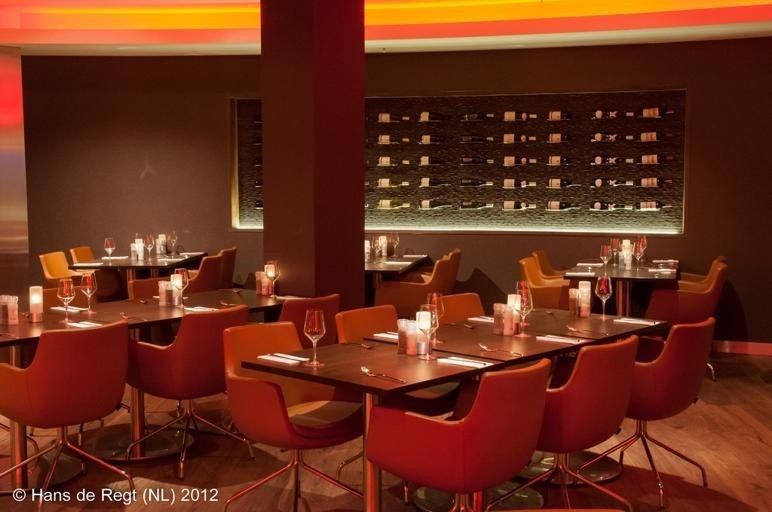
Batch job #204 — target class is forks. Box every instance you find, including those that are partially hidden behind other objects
[359,366,408,386]
[562,324,609,339]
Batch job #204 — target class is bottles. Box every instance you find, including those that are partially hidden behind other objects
[250,110,264,213]
[0,295,20,326]
[364,104,677,214]
[157,266,182,307]
[566,280,593,319]
[255,270,273,295]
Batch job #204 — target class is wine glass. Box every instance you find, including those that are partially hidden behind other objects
[597,236,649,276]
[56,277,77,325]
[303,306,329,368]
[364,229,402,265]
[395,289,448,361]
[593,274,616,320]
[101,229,179,261]
[492,279,538,339]
[174,268,190,303]
[266,259,281,298]
[77,272,99,316]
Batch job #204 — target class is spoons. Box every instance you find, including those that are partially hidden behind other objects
[477,339,524,358]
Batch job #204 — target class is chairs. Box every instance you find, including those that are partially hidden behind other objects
[0,239,733,511]
